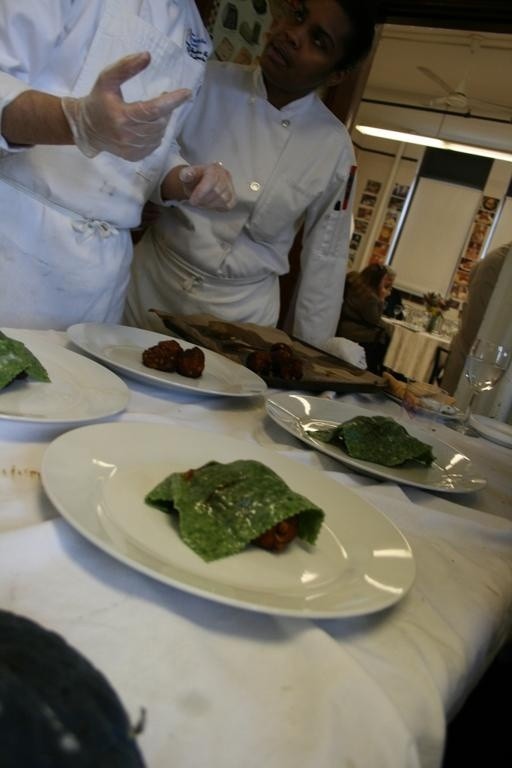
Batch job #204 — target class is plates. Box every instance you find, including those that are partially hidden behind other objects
[387,391,460,421]
[33,417,419,628]
[0,334,131,428]
[263,390,493,497]
[64,320,269,403]
[467,410,510,446]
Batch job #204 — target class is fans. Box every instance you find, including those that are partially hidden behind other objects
[411,51,512,125]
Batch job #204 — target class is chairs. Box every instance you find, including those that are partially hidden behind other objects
[340,313,391,377]
[428,343,450,384]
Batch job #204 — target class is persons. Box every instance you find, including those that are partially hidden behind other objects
[122,0,357,351]
[0,0,238,331]
[337,263,419,371]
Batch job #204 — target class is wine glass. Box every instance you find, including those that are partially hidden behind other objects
[444,336,511,438]
[379,300,459,340]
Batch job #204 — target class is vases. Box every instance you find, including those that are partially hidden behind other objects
[424,318,438,332]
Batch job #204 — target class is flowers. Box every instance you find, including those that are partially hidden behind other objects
[421,290,453,328]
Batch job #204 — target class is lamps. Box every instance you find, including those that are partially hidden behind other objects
[354,65,512,168]
